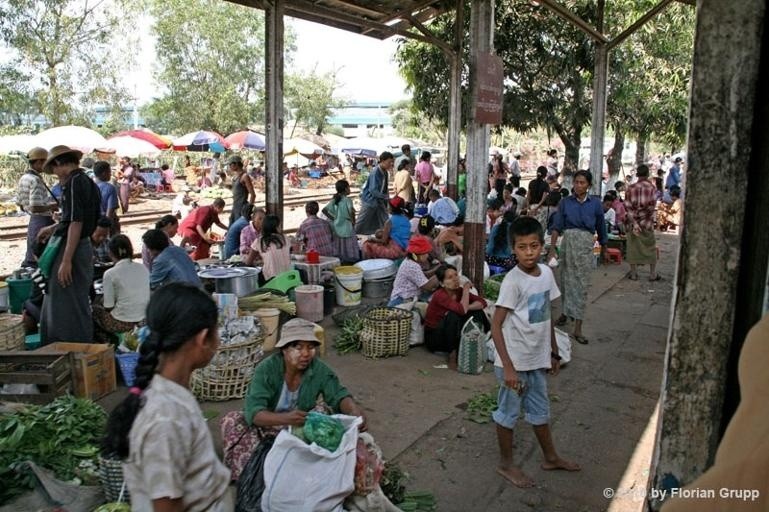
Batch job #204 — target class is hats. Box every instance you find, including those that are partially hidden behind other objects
[222,155,241,165]
[26,148,48,160]
[404,237,432,255]
[273,317,321,349]
[388,196,404,209]
[41,145,82,175]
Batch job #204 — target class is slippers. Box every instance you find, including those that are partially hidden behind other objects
[648,273,661,282]
[628,271,638,280]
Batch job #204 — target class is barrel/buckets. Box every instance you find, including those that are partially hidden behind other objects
[6,273,35,314]
[333,265,365,308]
[294,283,324,323]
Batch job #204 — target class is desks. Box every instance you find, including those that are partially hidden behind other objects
[289,253,340,292]
[607,232,628,255]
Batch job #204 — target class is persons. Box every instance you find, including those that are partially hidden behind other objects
[104,281,238,512]
[219,316,369,485]
[490,216,585,489]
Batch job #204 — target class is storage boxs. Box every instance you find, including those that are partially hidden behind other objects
[29,339,120,403]
[1,352,76,409]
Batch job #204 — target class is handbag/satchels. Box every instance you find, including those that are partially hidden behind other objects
[458,315,488,375]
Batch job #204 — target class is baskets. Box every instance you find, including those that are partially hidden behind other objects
[114,348,141,388]
[187,316,265,403]
[96,448,130,502]
[0,312,25,373]
[361,305,413,358]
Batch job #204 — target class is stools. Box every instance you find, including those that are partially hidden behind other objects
[603,247,622,264]
[655,246,659,261]
[157,185,164,192]
[165,185,172,192]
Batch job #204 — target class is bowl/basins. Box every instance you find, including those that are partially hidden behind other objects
[295,255,304,262]
